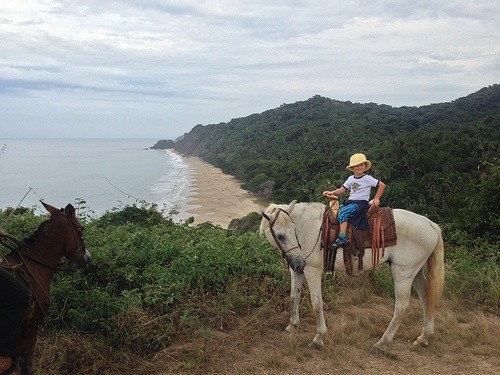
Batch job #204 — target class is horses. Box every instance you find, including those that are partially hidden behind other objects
[0,199,91,375]
[258,199,445,357]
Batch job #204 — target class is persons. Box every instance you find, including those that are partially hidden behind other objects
[323,152,386,248]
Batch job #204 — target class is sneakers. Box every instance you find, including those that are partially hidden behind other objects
[328,236,350,250]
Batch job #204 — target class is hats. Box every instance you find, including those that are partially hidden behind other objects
[346,153,371,172]
